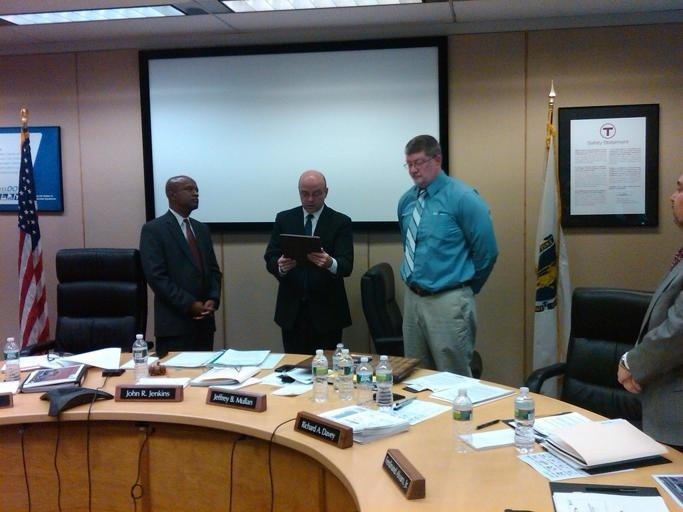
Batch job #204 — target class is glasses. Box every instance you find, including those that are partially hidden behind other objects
[404,158,436,167]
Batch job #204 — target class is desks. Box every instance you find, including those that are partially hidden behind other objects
[1,336,683,511]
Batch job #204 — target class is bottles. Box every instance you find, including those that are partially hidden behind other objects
[130,333,150,384]
[451,386,473,455]
[353,355,373,408]
[331,343,344,392]
[336,348,353,401]
[374,356,393,409]
[2,337,21,381]
[511,385,536,453]
[311,348,328,405]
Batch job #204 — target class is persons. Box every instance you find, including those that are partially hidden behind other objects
[395,136,498,378]
[262,170,353,356]
[138,174,223,352]
[617,177,682,454]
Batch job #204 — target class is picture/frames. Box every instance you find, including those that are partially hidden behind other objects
[359,258,486,377]
[18,245,154,356]
[554,102,660,233]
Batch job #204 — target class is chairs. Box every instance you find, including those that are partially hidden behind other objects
[522,283,657,431]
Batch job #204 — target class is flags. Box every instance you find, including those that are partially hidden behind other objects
[526,124,574,400]
[18,127,50,355]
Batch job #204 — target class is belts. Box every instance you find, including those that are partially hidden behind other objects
[406,282,472,296]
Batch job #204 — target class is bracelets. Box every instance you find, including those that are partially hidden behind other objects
[619,353,632,374]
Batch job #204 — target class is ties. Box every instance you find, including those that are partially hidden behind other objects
[304,213,314,237]
[398,189,427,282]
[184,219,203,272]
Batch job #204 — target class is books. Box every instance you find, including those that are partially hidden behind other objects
[21,349,671,511]
[278,234,320,268]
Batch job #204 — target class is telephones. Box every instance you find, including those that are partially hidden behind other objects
[40,387,114,418]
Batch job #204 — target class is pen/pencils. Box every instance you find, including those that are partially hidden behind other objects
[504,509,536,512]
[476,420,500,430]
[586,487,639,493]
[393,396,417,410]
